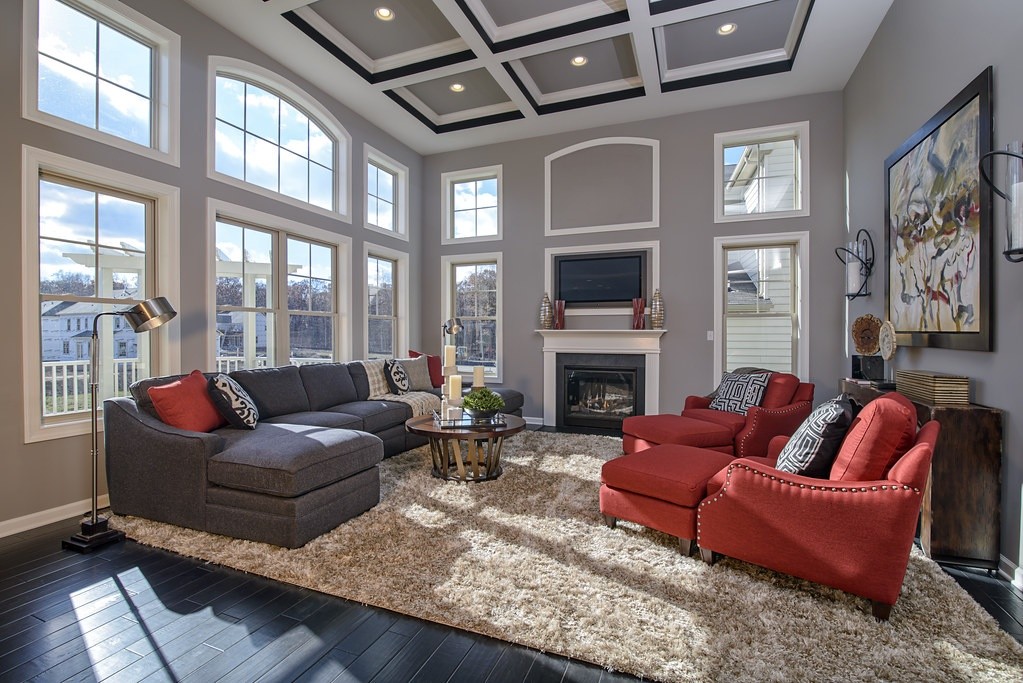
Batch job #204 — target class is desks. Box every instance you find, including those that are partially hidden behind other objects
[405,410,527,482]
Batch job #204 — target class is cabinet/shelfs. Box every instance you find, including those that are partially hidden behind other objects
[839,382,1004,575]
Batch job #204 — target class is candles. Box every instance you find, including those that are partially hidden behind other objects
[449,375,461,400]
[472,365,484,388]
[444,344,456,366]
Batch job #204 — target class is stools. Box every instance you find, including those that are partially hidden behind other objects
[599,443,737,557]
[621,413,735,457]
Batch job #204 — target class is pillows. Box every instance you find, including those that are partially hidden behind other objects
[147,370,227,433]
[774,392,854,473]
[207,373,259,429]
[711,370,775,416]
[383,359,411,395]
[408,348,443,388]
[395,353,434,390]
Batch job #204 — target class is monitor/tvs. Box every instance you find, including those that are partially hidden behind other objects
[554,251,647,309]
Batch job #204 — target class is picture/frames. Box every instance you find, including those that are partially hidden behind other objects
[883,63,994,354]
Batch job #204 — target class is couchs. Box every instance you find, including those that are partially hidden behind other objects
[103,361,524,549]
[681,369,819,457]
[695,389,940,620]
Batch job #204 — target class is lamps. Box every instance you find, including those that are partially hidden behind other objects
[978,140,1023,264]
[62,295,178,554]
[442,315,464,347]
[836,229,875,300]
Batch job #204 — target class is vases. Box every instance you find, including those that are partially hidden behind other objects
[553,299,567,329]
[650,288,665,330]
[537,291,552,328]
[632,297,646,330]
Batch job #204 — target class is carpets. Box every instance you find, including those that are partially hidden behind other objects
[104,424,1022,683]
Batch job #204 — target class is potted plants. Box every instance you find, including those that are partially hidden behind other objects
[460,388,505,418]
